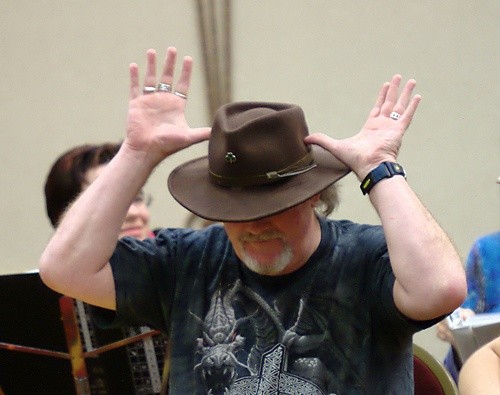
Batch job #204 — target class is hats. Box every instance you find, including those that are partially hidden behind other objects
[166,99,356,222]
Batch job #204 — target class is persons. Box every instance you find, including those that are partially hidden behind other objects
[437,176,500,395]
[40,46,467,395]
[44,140,151,239]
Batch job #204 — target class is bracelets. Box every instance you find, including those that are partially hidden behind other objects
[360,161,407,196]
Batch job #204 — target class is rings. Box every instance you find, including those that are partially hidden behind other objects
[175,92,188,99]
[390,112,401,120]
[157,83,173,92]
[143,87,155,92]
